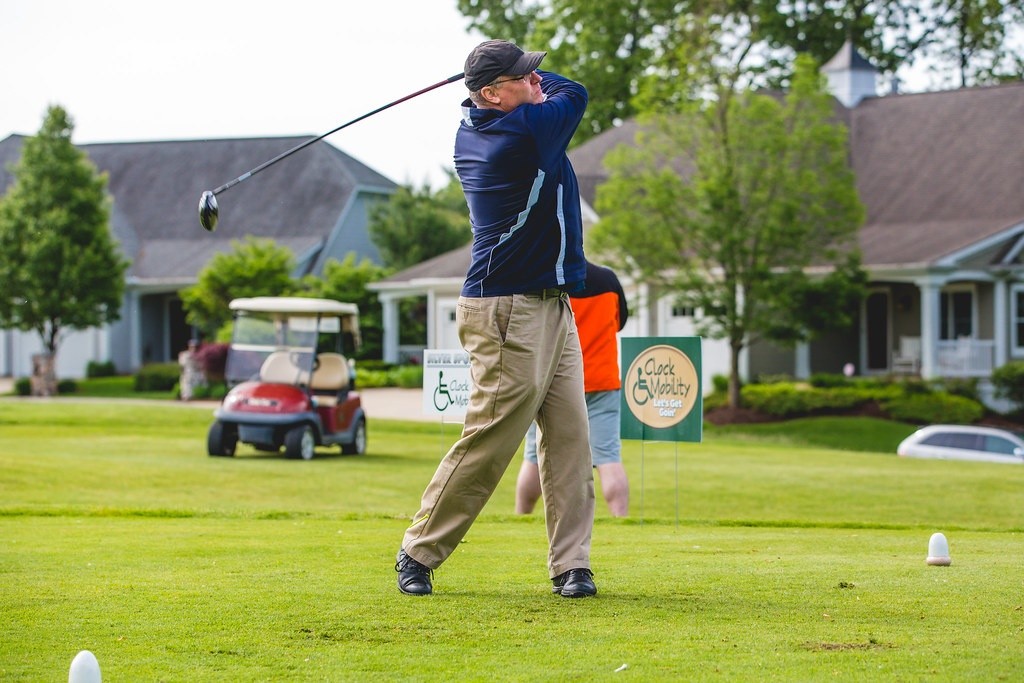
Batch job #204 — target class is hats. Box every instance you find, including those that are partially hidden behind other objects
[464,39,547,92]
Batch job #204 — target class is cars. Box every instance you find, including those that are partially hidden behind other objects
[895,423,1024,466]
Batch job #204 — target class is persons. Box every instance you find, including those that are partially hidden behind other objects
[514,255,630,520]
[391,38,600,601]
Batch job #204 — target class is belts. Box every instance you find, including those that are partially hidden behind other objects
[542,289,570,300]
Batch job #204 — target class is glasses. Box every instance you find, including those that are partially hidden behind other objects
[487,68,536,86]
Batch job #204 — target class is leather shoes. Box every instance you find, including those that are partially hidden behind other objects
[394,548,435,596]
[552,568,597,597]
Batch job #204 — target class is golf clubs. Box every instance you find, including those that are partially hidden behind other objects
[197,72,469,233]
